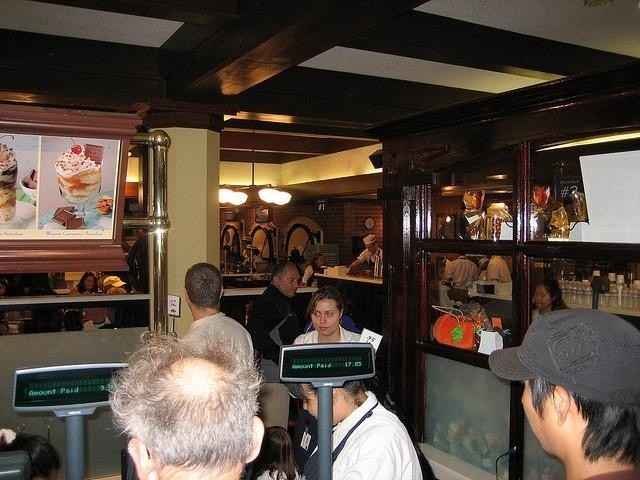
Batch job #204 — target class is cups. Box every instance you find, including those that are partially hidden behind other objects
[0,160,18,224]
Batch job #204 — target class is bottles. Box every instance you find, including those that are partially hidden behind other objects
[554,270,639,310]
[373,256,381,278]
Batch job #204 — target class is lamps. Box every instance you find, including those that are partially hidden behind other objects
[219,131,292,206]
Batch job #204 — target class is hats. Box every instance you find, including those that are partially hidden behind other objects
[103,275,128,288]
[363,234,377,245]
[488,309,639,409]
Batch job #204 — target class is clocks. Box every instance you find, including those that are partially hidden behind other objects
[364,216,376,230]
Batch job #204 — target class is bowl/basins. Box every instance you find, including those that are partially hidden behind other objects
[20,176,37,201]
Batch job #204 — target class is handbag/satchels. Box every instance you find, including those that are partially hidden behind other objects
[432,314,477,350]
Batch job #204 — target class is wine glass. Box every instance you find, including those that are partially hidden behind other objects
[58,168,103,230]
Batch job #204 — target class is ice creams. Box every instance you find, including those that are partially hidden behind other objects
[55,144,104,203]
[0,143,18,225]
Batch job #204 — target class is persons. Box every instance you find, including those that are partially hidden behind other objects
[300,253,325,286]
[240,244,261,273]
[347,233,383,277]
[279,379,427,480]
[305,285,362,339]
[244,260,307,387]
[288,281,365,345]
[483,254,512,284]
[0,426,61,480]
[105,328,267,479]
[530,276,571,323]
[289,247,305,265]
[176,261,256,366]
[243,424,304,480]
[487,306,640,480]
[444,253,479,290]
[2,269,150,335]
